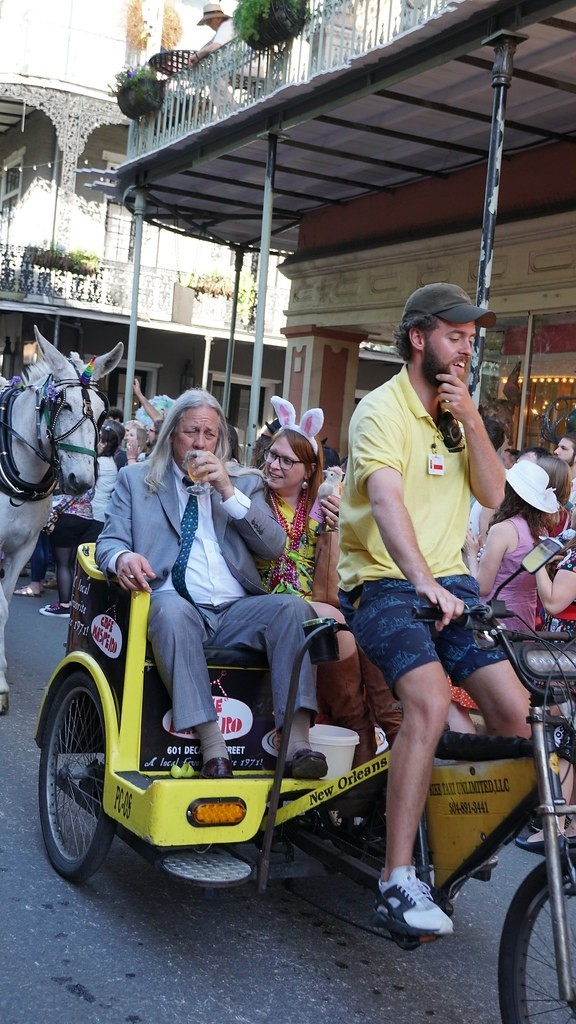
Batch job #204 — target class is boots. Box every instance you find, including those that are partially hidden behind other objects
[355,640,404,749]
[318,646,377,770]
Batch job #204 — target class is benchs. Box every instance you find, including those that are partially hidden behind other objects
[141,642,271,670]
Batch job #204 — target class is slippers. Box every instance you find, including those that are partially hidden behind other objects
[12,587,42,598]
[21,585,44,593]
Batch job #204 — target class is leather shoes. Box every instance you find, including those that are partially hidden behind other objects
[514,835,565,855]
[200,751,234,780]
[285,749,328,779]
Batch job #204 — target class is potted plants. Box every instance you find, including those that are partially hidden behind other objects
[172,270,257,331]
[233,0,311,50]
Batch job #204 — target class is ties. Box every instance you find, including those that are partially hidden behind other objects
[171,476,217,634]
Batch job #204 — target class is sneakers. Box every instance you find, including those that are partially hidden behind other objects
[372,865,453,935]
[39,604,70,618]
[481,855,498,871]
[44,601,64,609]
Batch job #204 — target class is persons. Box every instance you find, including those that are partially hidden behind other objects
[321,436,340,481]
[336,282,535,935]
[262,395,403,769]
[250,418,281,470]
[459,432,576,857]
[228,423,245,465]
[94,387,328,779]
[187,3,264,120]
[12,378,176,618]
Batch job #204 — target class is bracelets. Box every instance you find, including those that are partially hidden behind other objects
[195,52,201,59]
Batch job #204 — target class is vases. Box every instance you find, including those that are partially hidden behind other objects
[117,81,163,119]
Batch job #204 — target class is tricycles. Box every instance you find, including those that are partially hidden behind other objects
[33,536,575,1024]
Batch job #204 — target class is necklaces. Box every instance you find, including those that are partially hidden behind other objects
[476,544,486,562]
[262,487,311,593]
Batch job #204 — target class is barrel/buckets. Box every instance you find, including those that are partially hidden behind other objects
[309,724,360,781]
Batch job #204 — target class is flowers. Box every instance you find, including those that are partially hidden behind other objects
[106,61,157,96]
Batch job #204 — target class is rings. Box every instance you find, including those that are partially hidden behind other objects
[127,574,135,579]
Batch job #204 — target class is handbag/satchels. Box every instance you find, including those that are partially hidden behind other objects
[312,525,341,608]
[42,510,59,534]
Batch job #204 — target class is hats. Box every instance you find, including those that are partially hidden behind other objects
[504,459,560,514]
[322,446,341,469]
[402,283,497,328]
[265,418,282,434]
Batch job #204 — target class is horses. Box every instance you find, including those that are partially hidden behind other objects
[0,324,125,713]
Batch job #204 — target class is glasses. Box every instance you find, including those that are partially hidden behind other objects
[149,428,157,433]
[263,446,305,471]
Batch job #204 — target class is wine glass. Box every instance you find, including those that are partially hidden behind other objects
[185,451,207,496]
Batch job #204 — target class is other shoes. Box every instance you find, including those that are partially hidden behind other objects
[42,578,58,588]
[18,572,29,577]
